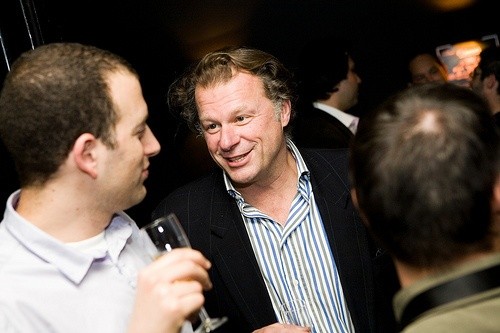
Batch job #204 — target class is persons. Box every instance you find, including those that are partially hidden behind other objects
[180,44,500,333]
[0,43,213,333]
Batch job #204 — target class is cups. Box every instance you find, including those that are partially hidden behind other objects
[279,299,314,333]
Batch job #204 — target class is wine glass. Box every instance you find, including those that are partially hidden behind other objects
[139,213,227,333]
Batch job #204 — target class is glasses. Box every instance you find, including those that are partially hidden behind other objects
[469,70,484,79]
